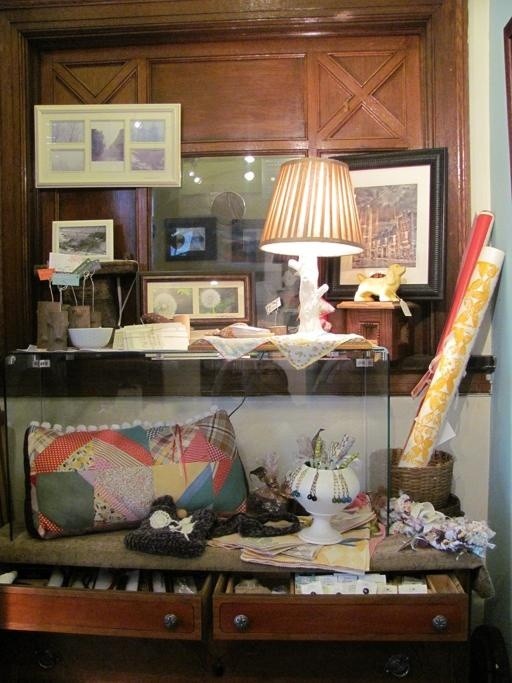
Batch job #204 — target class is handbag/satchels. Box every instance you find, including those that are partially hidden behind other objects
[124,497,301,559]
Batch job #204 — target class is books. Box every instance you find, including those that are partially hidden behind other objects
[210,509,373,575]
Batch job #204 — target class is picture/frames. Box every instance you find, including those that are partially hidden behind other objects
[53,220,112,261]
[167,219,216,260]
[137,270,256,325]
[231,219,299,262]
[324,147,447,300]
[35,103,182,188]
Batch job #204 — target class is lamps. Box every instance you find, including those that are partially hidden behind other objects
[260,158,363,332]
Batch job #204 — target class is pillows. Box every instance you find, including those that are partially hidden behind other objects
[24,406,248,542]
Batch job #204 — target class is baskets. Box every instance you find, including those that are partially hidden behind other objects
[390,446,455,509]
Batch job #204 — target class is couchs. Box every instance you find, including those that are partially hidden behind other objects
[1,515,494,677]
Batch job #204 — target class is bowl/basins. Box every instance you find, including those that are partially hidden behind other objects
[68,327,114,350]
